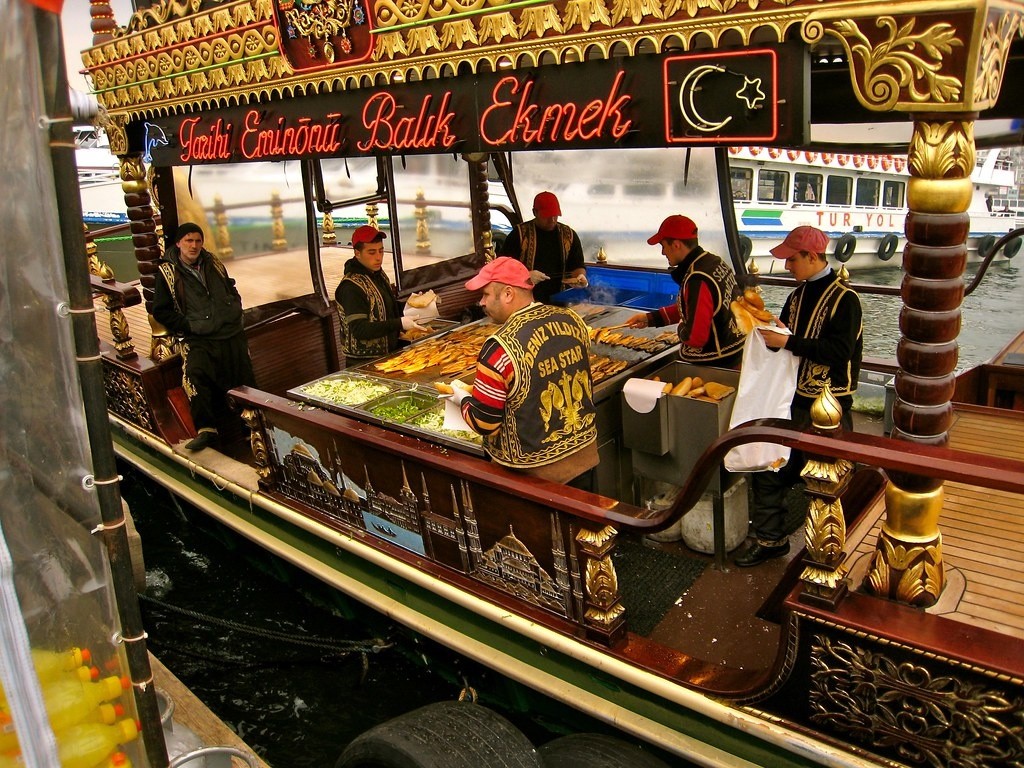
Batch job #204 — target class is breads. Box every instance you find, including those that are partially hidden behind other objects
[434,382,474,394]
[650,376,736,402]
[730,291,773,334]
[407,290,436,307]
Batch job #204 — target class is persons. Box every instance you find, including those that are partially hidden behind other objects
[335,225,427,368]
[444,257,599,493]
[986,195,993,211]
[732,226,863,567]
[497,191,589,303]
[998,206,1016,217]
[624,214,745,370]
[1003,156,1012,169]
[152,223,261,451]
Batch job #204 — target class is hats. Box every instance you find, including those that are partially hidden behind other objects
[533,191,562,219]
[647,214,697,245]
[175,223,204,243]
[352,225,387,248]
[464,256,535,291]
[769,226,830,259]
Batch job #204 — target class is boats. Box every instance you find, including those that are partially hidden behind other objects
[69,9,1024,768]
[497,144,1017,273]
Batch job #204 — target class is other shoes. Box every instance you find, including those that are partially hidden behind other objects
[734,541,790,567]
[185,431,218,450]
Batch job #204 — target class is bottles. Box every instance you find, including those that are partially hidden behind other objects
[0,645,143,768]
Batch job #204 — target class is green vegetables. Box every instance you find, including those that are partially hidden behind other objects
[369,392,437,425]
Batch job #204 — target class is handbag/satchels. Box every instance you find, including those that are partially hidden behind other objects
[723,326,800,472]
